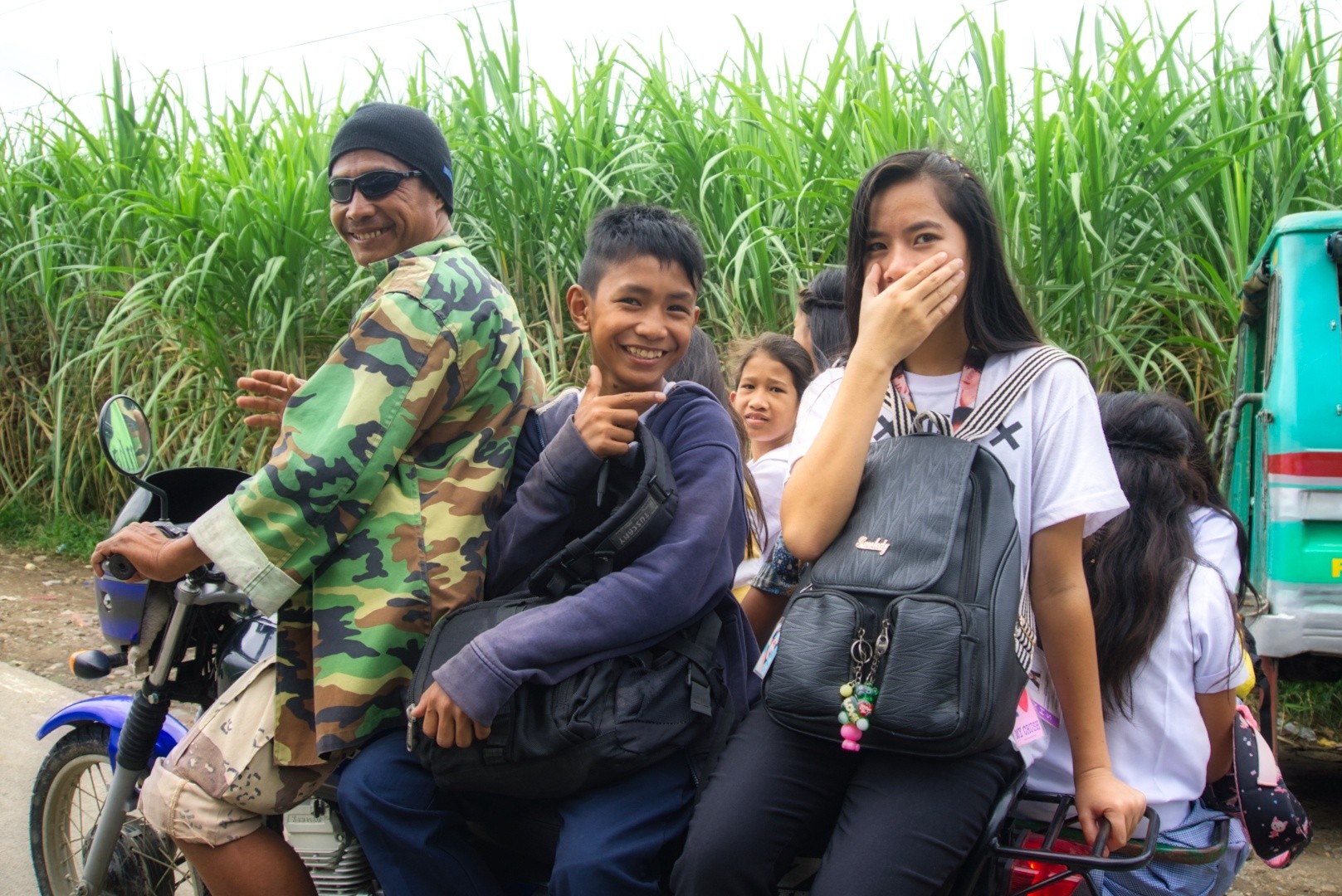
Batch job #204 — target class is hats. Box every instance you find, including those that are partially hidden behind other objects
[329,102,453,216]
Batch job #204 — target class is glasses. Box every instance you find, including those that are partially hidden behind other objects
[329,170,425,203]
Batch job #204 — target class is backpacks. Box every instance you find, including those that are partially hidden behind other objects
[764,349,1088,757]
[408,389,733,796]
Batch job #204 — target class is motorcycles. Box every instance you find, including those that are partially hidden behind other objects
[29,395,1231,896]
[1208,208,1342,679]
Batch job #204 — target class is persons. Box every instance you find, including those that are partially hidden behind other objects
[337,206,762,896]
[1008,396,1256,896]
[88,104,545,896]
[672,151,1130,896]
[727,270,856,641]
